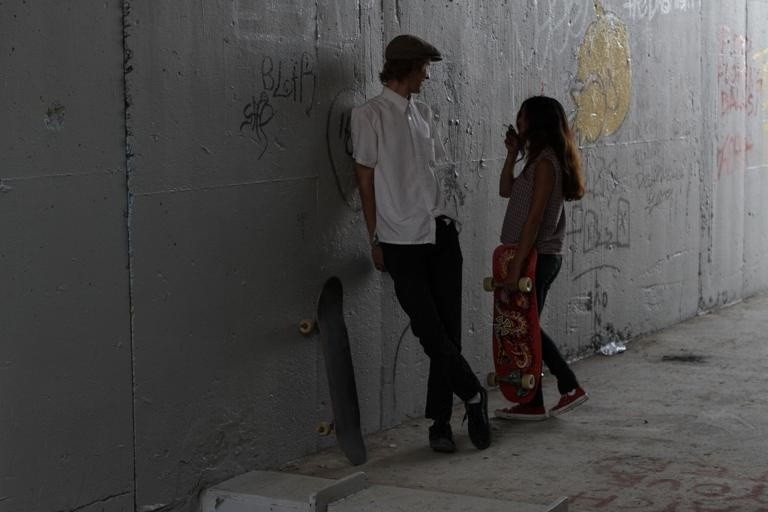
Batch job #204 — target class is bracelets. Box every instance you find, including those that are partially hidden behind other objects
[370,239,379,247]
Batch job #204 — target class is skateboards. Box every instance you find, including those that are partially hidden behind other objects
[484,242,541,402]
[298,275,366,465]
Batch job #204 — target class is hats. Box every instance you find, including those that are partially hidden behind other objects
[385,35,443,61]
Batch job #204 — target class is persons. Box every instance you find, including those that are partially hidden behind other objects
[493,95,590,422]
[350,34,491,452]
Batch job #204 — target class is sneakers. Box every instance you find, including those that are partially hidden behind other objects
[550,388,589,416]
[496,405,546,420]
[463,388,490,449]
[429,424,455,452]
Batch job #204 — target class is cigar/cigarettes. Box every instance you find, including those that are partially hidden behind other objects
[503,124,515,130]
